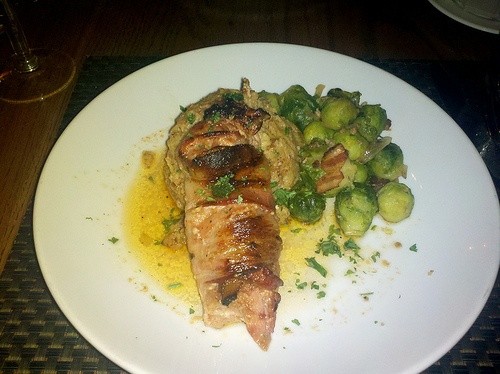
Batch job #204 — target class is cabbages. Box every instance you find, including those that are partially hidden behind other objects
[264,84,415,238]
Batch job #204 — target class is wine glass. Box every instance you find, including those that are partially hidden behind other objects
[0,0,75,104]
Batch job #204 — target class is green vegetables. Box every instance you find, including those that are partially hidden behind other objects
[177,91,382,299]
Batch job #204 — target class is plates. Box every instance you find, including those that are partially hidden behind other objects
[428,0,500,34]
[33,42,500,374]
